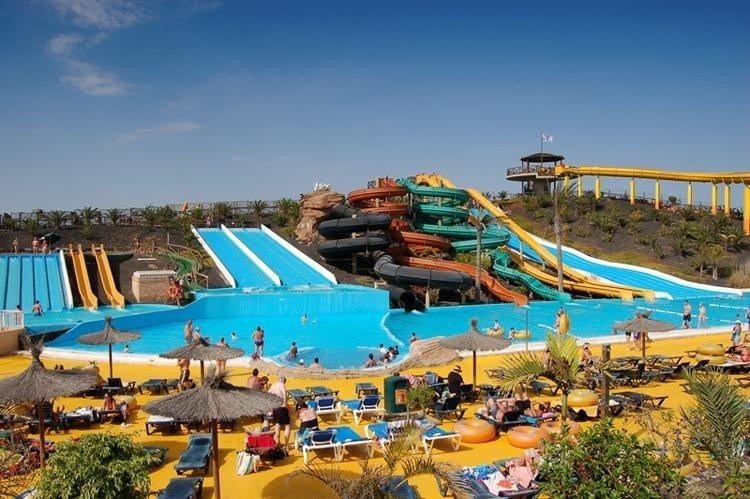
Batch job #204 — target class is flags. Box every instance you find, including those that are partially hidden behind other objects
[542,135,556,144]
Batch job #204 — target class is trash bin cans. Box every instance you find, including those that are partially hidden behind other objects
[384,376,409,414]
[602,344,611,362]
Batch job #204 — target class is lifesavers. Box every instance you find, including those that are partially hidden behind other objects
[567,388,598,407]
[515,329,528,339]
[455,418,496,443]
[507,422,580,449]
[487,326,504,336]
[696,344,725,364]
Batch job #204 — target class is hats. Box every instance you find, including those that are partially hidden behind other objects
[453,366,462,372]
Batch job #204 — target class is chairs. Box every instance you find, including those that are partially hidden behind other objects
[74,375,194,399]
[455,471,503,499]
[493,457,577,486]
[355,383,379,399]
[337,396,381,425]
[245,435,277,465]
[1,400,237,434]
[326,426,374,462]
[378,476,418,498]
[470,464,538,499]
[175,434,213,475]
[286,389,311,405]
[158,477,203,499]
[396,345,749,432]
[305,386,339,401]
[295,428,340,467]
[304,396,342,425]
[415,420,462,455]
[365,423,421,458]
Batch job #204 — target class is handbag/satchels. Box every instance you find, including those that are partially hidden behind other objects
[235,451,261,476]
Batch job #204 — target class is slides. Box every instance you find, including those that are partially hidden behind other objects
[400,178,573,303]
[93,243,125,308]
[317,201,474,309]
[556,166,749,184]
[431,176,654,300]
[347,177,529,306]
[416,176,633,301]
[221,222,339,289]
[69,244,98,308]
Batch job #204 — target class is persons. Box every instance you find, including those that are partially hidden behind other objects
[32,237,40,254]
[41,240,49,253]
[206,214,212,228]
[13,237,19,253]
